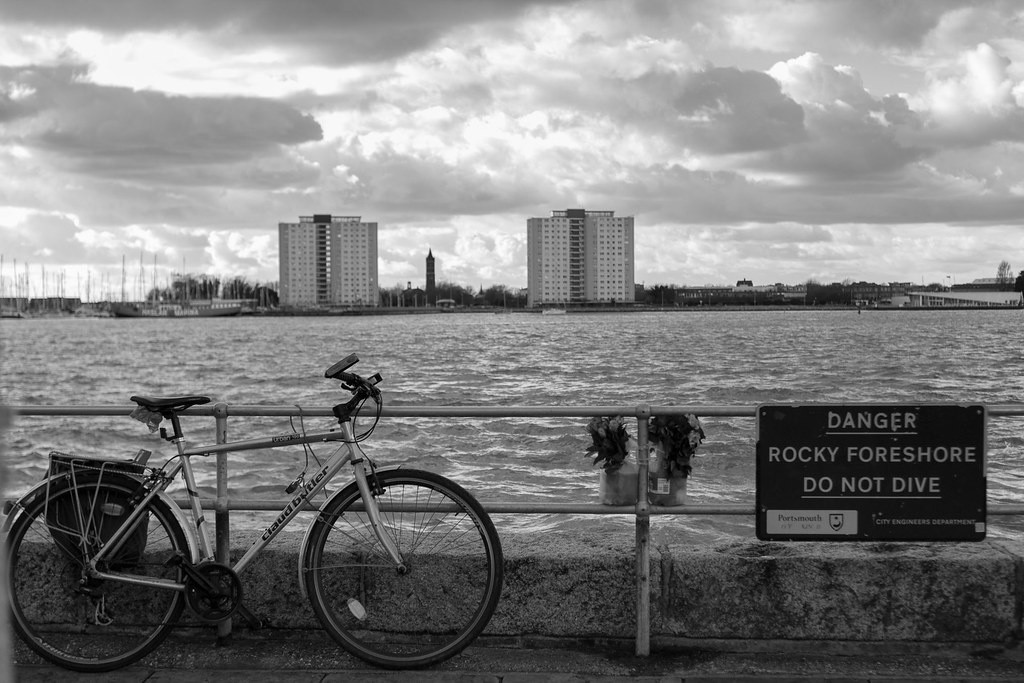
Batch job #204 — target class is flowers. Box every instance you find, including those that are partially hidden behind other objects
[653,417,701,456]
[593,417,631,464]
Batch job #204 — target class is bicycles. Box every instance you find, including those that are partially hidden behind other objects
[1,351,504,670]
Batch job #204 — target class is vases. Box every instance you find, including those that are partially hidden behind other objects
[600,466,639,505]
[650,463,689,505]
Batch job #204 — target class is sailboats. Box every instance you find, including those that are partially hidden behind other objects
[0,253,114,319]
[494,293,511,314]
[111,253,242,318]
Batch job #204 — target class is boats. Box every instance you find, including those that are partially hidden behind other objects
[440,308,455,314]
[540,308,566,315]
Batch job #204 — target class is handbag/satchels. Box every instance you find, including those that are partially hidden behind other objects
[35,455,149,563]
